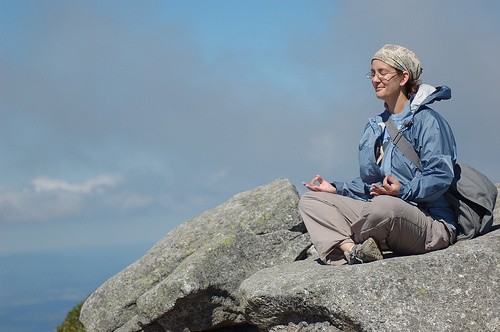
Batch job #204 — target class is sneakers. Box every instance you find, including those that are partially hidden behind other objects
[348,237,382,263]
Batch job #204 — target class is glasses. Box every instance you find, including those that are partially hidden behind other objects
[366,71,398,77]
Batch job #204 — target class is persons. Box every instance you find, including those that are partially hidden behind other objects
[298,43,461,265]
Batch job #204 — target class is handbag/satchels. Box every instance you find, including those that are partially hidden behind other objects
[447,161,499,240]
